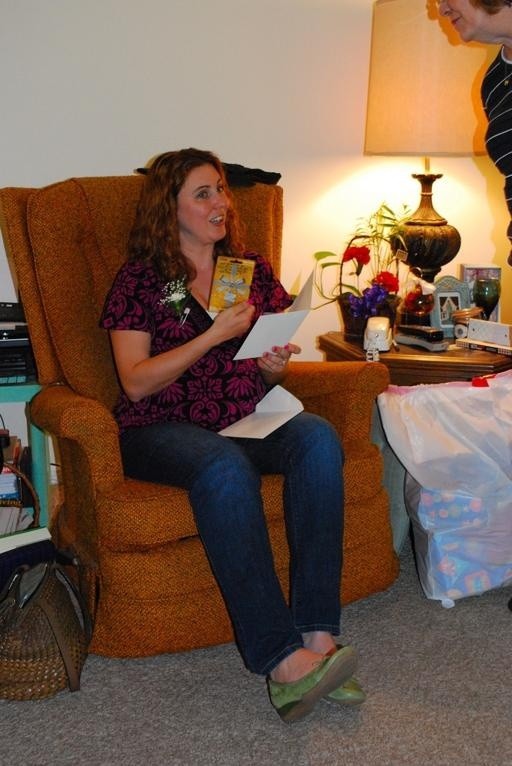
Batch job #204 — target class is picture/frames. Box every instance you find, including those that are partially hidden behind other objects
[430,274,471,338]
[461,263,502,322]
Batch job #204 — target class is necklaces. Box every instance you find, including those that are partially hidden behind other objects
[504,61,512,86]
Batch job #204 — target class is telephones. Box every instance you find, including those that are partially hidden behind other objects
[362,317,393,362]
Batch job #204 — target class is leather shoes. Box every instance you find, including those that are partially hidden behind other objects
[265,642,357,723]
[324,677,364,708]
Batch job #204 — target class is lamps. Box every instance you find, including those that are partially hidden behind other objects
[362,0,503,284]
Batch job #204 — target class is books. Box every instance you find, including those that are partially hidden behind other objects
[0,526,52,557]
[456,337,512,356]
[3,507,16,534]
[10,507,20,533]
[0,436,21,499]
[0,507,10,534]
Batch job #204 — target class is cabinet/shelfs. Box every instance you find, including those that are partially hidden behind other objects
[0,301,53,557]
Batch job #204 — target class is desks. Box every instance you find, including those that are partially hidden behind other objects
[317,329,512,385]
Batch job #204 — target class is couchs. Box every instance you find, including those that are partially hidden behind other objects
[0,172,402,661]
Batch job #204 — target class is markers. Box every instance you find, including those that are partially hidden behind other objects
[393,339,400,351]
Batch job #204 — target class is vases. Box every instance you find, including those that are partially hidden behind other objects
[336,292,402,344]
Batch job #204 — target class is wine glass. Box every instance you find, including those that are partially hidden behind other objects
[473,276,502,320]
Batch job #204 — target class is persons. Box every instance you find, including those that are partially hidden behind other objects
[433,0,512,263]
[96,146,367,726]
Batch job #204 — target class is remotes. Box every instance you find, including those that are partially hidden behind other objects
[397,324,444,342]
[395,334,449,351]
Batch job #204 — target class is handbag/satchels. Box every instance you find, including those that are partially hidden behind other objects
[1,461,99,700]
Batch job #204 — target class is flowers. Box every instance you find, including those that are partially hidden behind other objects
[306,199,412,319]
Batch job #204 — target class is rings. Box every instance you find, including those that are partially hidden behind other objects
[282,359,287,367]
